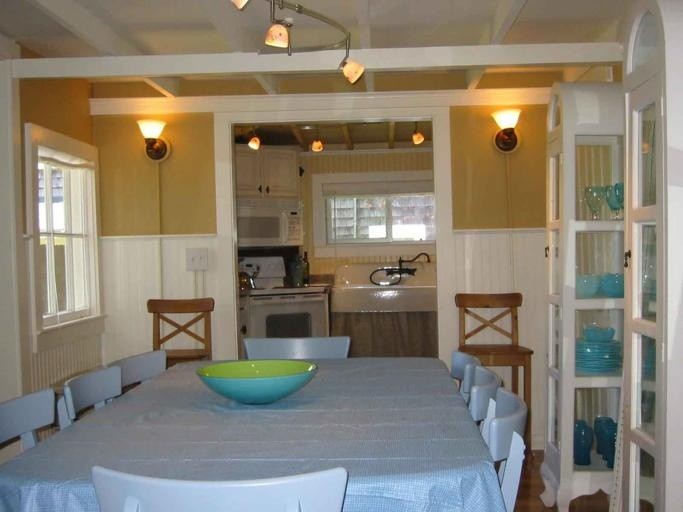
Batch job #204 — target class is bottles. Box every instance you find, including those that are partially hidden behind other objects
[302,250,311,283]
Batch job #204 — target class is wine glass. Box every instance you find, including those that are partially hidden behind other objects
[584,183,625,220]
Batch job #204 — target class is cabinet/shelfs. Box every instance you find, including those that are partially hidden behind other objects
[540,81,626,512]
[625,1,683,511]
[235,143,299,199]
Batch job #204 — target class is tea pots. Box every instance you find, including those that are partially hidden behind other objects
[237,259,259,290]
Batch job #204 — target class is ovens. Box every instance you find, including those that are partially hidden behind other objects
[239,293,330,337]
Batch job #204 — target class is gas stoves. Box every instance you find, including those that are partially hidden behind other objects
[240,283,329,297]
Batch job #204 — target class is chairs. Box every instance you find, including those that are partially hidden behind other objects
[0,350,170,447]
[450,350,528,512]
[88,466,347,512]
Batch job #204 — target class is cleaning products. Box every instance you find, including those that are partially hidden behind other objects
[291,250,309,287]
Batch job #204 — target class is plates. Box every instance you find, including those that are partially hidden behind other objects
[575,325,625,373]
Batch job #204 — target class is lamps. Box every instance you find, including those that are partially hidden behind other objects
[311,127,324,152]
[230,0,366,86]
[412,121,425,145]
[248,130,260,150]
[135,120,170,161]
[491,109,522,153]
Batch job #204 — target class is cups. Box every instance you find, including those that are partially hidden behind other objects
[576,416,615,470]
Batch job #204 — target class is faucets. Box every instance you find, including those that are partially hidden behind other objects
[398,251,431,271]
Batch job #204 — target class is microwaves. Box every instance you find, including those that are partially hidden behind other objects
[237,207,303,247]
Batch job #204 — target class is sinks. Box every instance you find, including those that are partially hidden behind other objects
[330,279,438,312]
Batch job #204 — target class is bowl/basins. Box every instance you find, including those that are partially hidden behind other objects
[576,272,626,298]
[194,359,318,405]
